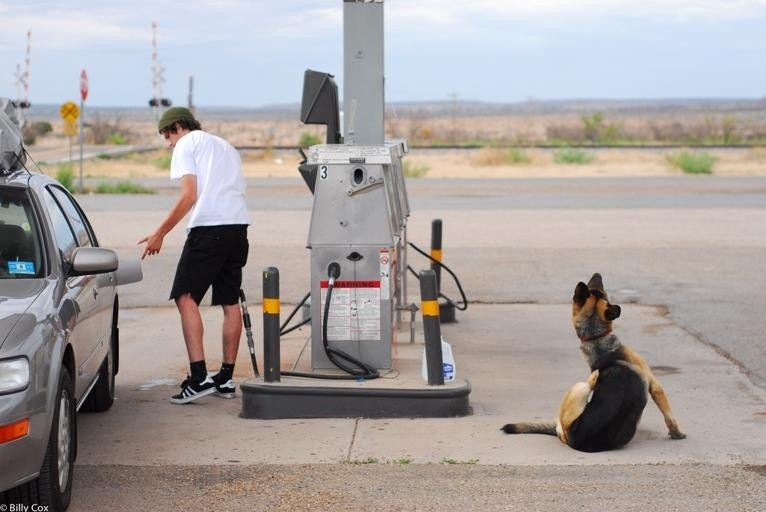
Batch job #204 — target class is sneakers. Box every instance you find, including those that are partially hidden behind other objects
[170,375,236,404]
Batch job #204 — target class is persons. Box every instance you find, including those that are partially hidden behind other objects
[136,107,251,404]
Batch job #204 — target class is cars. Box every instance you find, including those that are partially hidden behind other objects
[0,170,143,512]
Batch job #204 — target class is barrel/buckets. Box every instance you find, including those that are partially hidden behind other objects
[423,335,455,383]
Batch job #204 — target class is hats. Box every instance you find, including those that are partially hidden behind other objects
[158,107,193,135]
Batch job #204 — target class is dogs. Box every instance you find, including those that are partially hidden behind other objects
[500,272,687,452]
